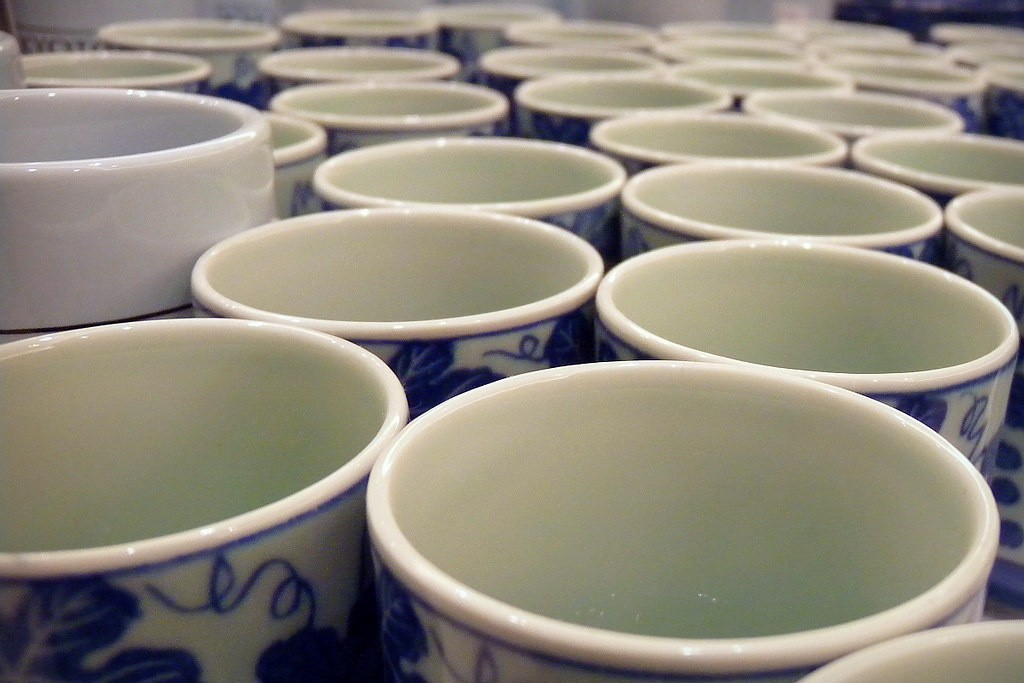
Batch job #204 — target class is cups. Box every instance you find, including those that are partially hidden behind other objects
[668,61,854,114]
[622,162,945,266]
[269,82,509,159]
[0,90,278,345]
[780,21,984,134]
[191,208,605,423]
[929,22,1024,141]
[851,133,1024,211]
[281,9,440,51]
[743,89,965,148]
[943,187,1024,608]
[363,360,1000,683]
[591,239,1020,485]
[0,0,303,111]
[313,134,627,250]
[264,112,327,221]
[513,73,733,152]
[0,319,411,683]
[478,45,664,136]
[659,23,822,69]
[503,18,656,54]
[426,4,561,84]
[587,114,849,183]
[261,46,461,91]
[796,620,1024,683]
